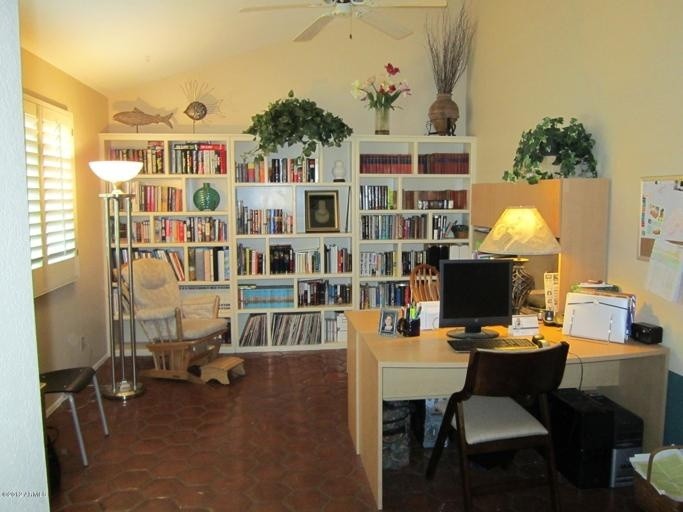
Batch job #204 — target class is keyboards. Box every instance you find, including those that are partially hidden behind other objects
[446,338,537,354]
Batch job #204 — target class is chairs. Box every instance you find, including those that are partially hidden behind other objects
[40,367,111,468]
[410,263,440,304]
[425,341,569,512]
[119,258,229,385]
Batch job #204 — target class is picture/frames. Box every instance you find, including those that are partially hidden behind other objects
[304,190,339,233]
[378,308,398,336]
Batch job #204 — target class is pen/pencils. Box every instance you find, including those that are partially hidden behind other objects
[400,299,422,319]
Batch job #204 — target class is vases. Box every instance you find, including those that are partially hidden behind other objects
[425,92,460,136]
[375,107,390,135]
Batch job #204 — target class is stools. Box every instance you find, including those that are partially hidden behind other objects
[200,357,246,385]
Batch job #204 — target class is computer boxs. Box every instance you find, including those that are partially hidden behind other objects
[549,386,615,492]
[587,392,645,488]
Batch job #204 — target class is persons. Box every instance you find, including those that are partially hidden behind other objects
[109,140,165,173]
[382,313,395,332]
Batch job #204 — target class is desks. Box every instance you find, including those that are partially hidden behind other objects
[360,321,671,510]
[343,307,533,455]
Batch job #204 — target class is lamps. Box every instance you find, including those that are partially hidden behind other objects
[88,161,146,400]
[478,204,562,316]
[336,3,353,39]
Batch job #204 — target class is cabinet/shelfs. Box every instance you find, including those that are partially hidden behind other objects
[99,132,235,354]
[230,133,355,351]
[356,135,476,309]
[471,178,609,316]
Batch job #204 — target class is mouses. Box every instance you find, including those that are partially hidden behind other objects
[537,339,549,348]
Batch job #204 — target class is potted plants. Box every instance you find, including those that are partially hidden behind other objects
[504,116,597,183]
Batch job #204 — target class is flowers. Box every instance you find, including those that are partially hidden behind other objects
[351,61,416,113]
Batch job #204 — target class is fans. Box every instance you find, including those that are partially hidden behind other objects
[240,0,447,42]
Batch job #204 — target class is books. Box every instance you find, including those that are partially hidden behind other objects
[107,180,183,213]
[168,143,227,178]
[441,242,470,261]
[418,151,470,174]
[297,280,353,308]
[325,312,348,344]
[235,159,316,183]
[324,244,352,274]
[401,189,468,210]
[359,281,413,310]
[359,214,429,241]
[359,250,398,277]
[238,283,294,309]
[188,247,230,282]
[154,218,228,244]
[178,285,232,316]
[113,286,126,322]
[110,220,149,243]
[360,153,414,174]
[401,243,441,277]
[543,272,559,316]
[272,313,321,346]
[237,246,264,275]
[358,184,398,209]
[562,279,636,345]
[113,249,184,283]
[239,314,268,345]
[269,242,320,274]
[236,200,293,234]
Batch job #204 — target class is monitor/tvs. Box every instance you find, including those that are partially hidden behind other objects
[438,259,512,339]
